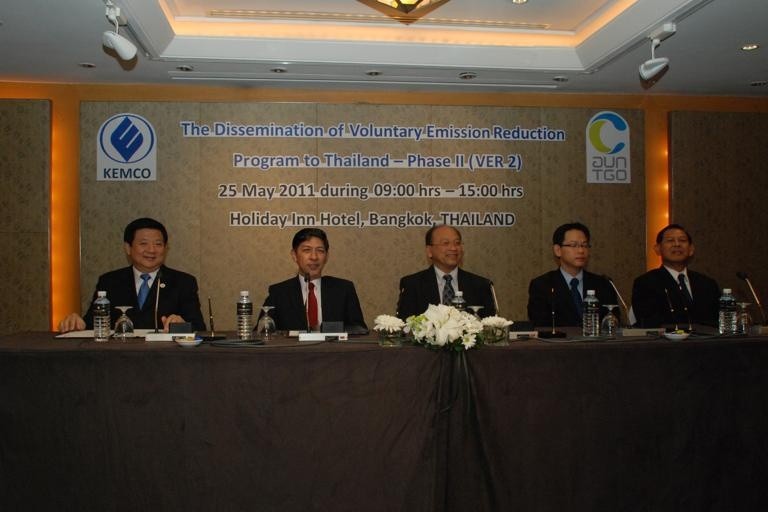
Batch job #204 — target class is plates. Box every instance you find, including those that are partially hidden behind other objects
[664,332,690,341]
[176,339,204,348]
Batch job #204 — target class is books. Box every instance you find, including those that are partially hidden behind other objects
[53,327,164,339]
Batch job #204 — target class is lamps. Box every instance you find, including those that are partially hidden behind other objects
[638,21,676,80]
[99,5,137,60]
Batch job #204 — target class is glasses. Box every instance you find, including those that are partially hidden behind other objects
[561,242,592,248]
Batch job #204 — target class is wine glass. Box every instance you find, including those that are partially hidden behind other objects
[601,304,620,340]
[257,306,277,342]
[470,306,484,321]
[115,305,137,345]
[738,302,754,335]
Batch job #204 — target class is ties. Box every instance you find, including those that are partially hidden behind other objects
[304,283,318,331]
[443,274,455,306]
[678,273,693,304]
[137,273,150,311]
[569,278,583,326]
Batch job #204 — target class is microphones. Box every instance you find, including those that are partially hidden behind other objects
[305,272,311,333]
[736,270,767,326]
[148,270,163,334]
[602,274,632,329]
[488,279,500,317]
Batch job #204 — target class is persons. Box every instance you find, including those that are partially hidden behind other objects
[632,223,720,327]
[397,224,498,331]
[253,228,367,335]
[59,218,206,336]
[528,222,618,328]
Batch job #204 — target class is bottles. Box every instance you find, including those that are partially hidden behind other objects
[452,291,466,311]
[236,291,253,340]
[94,290,111,343]
[582,290,600,339]
[718,287,738,337]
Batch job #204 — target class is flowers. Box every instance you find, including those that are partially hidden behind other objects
[371,297,513,354]
